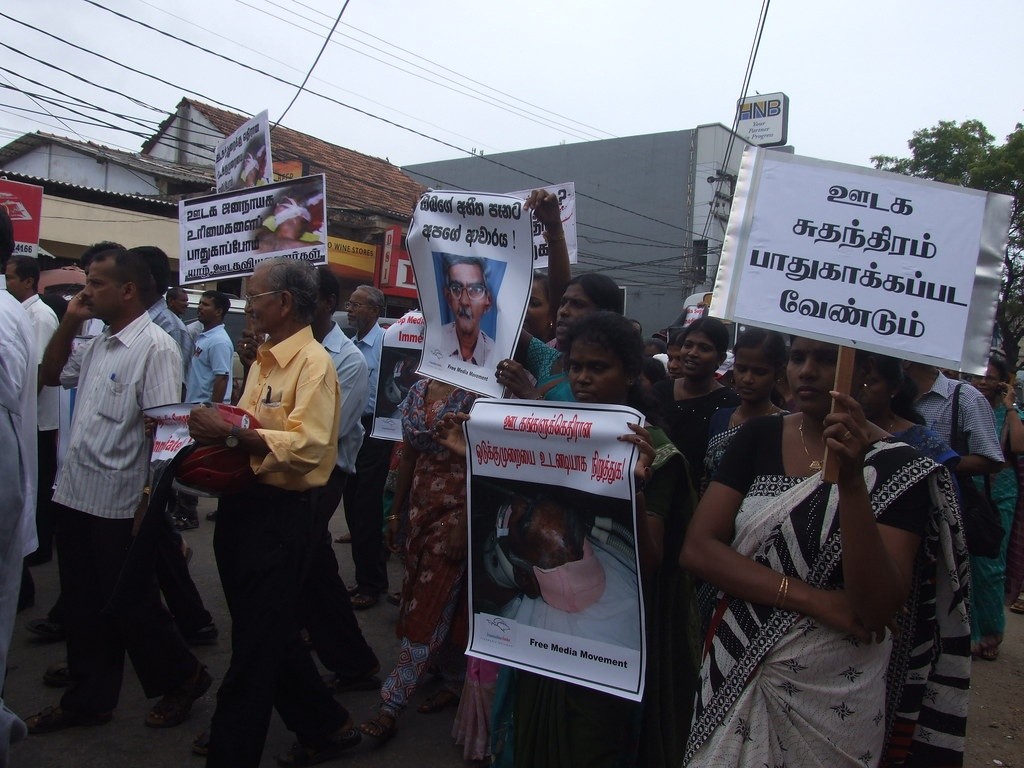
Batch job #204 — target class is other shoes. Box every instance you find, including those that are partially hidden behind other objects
[349,580,389,609]
[172,515,199,531]
[207,511,217,520]
[386,592,401,606]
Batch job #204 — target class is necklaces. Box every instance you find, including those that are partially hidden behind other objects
[430,385,451,413]
[888,414,896,432]
[732,400,773,426]
[799,418,824,471]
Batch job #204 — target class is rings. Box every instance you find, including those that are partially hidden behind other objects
[503,362,508,368]
[839,431,851,441]
[244,344,247,348]
[500,371,502,373]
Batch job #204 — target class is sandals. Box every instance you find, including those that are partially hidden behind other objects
[26,617,66,638]
[43,661,72,687]
[1010,593,1024,614]
[145,673,212,728]
[359,712,395,737]
[416,690,448,713]
[979,643,999,660]
[22,704,113,733]
[181,611,218,641]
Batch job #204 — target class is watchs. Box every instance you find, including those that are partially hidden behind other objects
[225,425,241,447]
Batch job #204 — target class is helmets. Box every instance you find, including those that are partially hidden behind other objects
[175,402,263,498]
[484,498,606,613]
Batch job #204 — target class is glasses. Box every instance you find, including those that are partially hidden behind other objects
[449,284,486,300]
[244,289,282,309]
[344,301,368,310]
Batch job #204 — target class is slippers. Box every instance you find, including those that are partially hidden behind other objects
[191,730,210,755]
[320,674,381,695]
[277,729,361,768]
[182,545,193,564]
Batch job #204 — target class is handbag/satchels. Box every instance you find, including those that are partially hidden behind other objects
[961,495,1005,559]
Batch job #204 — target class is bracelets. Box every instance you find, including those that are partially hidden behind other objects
[543,232,565,243]
[535,396,545,400]
[144,487,150,495]
[778,576,788,608]
[774,576,786,608]
[1006,406,1019,413]
[386,515,401,520]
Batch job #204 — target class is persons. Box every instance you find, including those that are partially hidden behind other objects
[0,190,697,768]
[257,197,323,253]
[678,335,972,768]
[638,317,1024,660]
[439,256,494,366]
[472,492,606,614]
